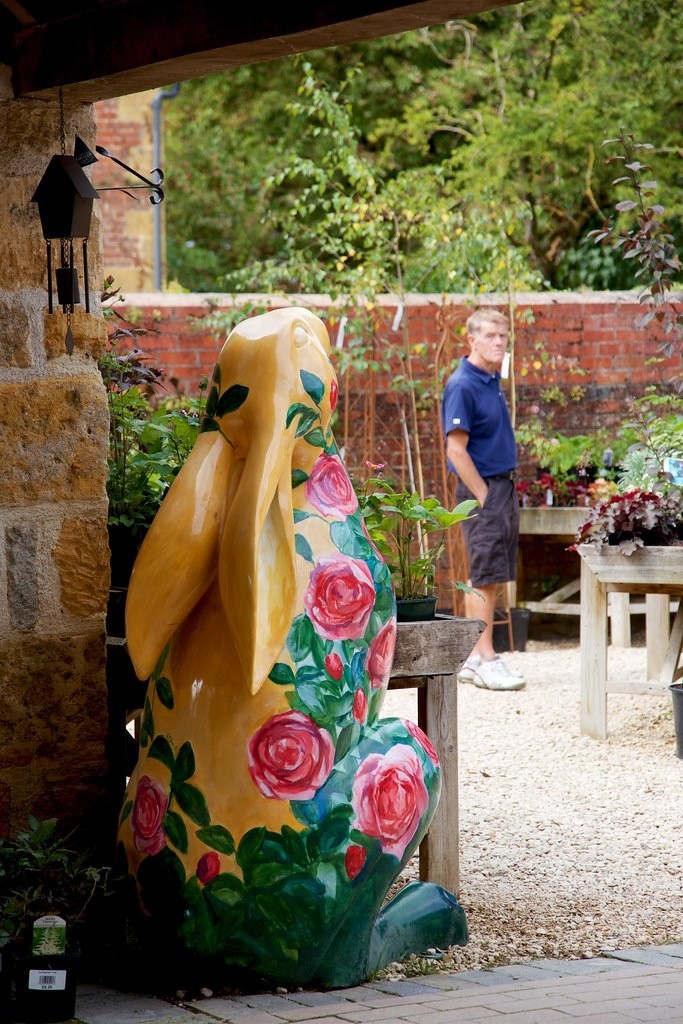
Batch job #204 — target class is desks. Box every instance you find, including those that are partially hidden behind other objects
[510,506,683,648]
[365,613,489,897]
[576,543,683,739]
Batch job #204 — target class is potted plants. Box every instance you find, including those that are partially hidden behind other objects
[0,814,118,1024]
[349,473,481,622]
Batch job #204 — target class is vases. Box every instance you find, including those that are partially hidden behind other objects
[667,684,683,761]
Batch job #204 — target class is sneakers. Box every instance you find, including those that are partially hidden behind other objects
[457,659,478,683]
[473,658,526,689]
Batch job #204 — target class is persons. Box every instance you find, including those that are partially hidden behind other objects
[440,309,526,691]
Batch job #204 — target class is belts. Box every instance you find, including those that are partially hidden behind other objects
[489,470,516,480]
